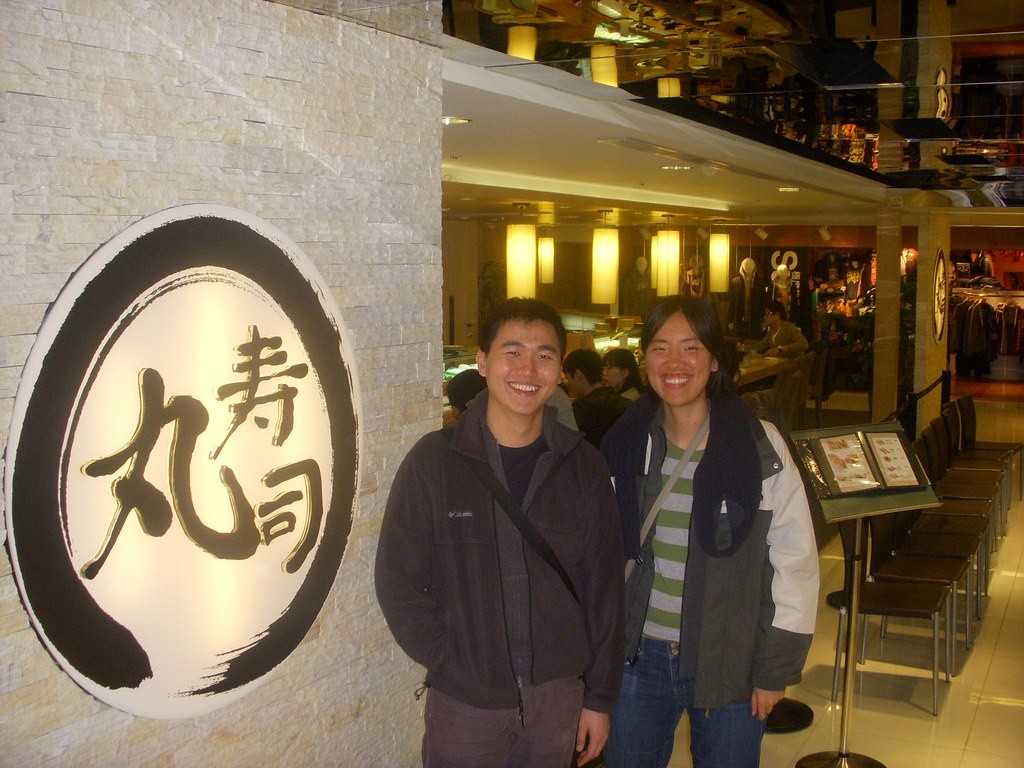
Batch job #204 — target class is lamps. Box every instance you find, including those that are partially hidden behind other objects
[657,78,680,98]
[591,45,618,88]
[819,225,831,241]
[508,27,536,60]
[506,203,730,304]
[754,226,768,240]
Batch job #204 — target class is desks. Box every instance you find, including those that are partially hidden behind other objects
[737,355,789,387]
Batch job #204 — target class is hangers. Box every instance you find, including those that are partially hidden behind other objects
[962,295,1015,307]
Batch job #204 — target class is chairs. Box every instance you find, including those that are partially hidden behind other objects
[826,393,1024,716]
[741,348,828,432]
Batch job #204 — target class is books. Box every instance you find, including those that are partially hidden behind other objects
[807,429,929,497]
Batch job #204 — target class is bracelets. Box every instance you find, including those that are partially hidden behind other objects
[777,344,782,352]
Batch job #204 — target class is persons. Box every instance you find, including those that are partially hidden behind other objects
[727,256,764,338]
[622,257,648,315]
[974,249,992,278]
[600,294,819,768]
[446,370,488,417]
[601,349,644,401]
[563,349,634,449]
[737,301,808,359]
[545,386,578,432]
[814,253,865,299]
[766,264,801,331]
[683,265,710,298]
[374,296,626,768]
